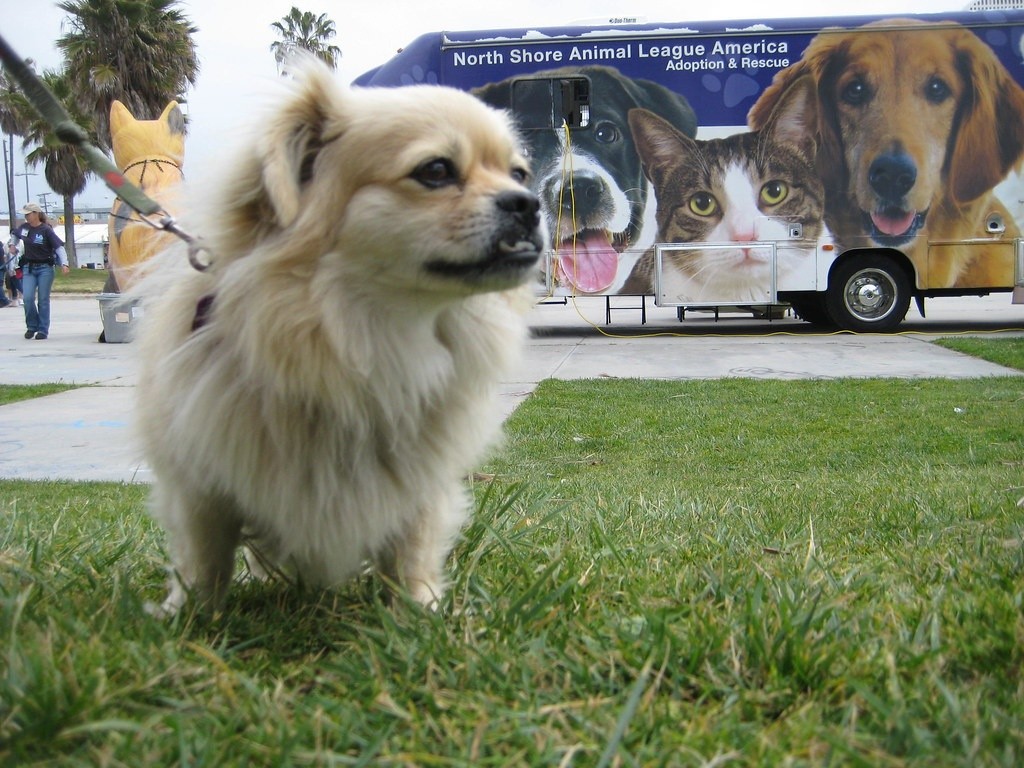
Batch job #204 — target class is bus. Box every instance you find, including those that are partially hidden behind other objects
[345,0,1024,332]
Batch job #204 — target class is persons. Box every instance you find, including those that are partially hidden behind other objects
[0,227,24,307]
[10,202,70,340]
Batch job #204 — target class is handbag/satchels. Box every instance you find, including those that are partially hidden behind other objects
[14,268,23,279]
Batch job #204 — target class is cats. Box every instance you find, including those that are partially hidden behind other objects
[100,94,203,304]
[625,72,840,307]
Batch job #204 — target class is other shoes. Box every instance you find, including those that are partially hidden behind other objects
[8,301,19,307]
[35,333,47,339]
[25,330,36,339]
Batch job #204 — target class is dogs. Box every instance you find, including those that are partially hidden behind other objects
[740,15,1024,299]
[456,64,704,308]
[126,56,555,635]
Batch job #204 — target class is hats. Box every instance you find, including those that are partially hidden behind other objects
[16,203,41,214]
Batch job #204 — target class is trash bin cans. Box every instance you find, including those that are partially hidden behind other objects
[95,293,143,343]
